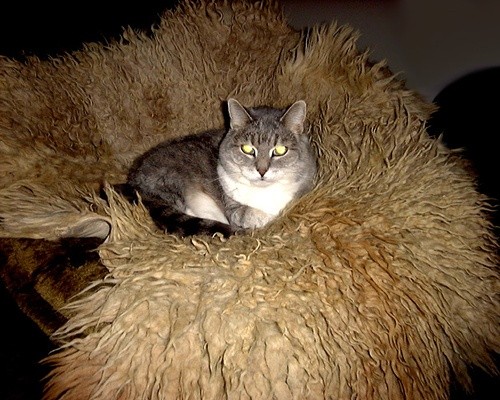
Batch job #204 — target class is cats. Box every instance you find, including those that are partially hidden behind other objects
[101,98,318,240]
